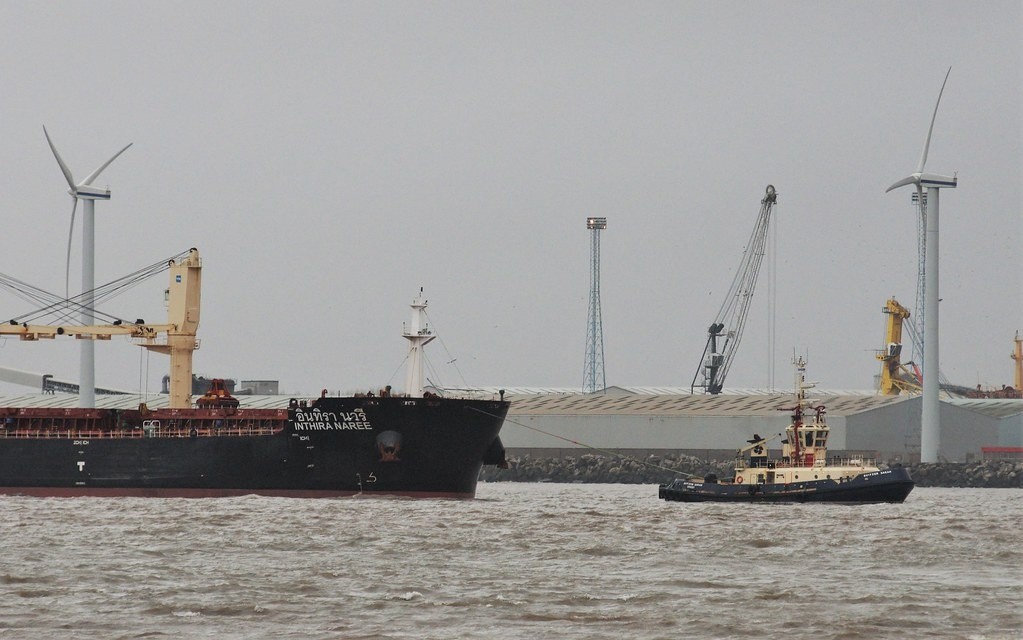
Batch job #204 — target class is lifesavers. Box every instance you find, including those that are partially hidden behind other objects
[737,476,743,484]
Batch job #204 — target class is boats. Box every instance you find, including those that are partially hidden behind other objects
[658,357,915,505]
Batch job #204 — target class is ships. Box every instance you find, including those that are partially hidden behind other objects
[1,377,514,505]
